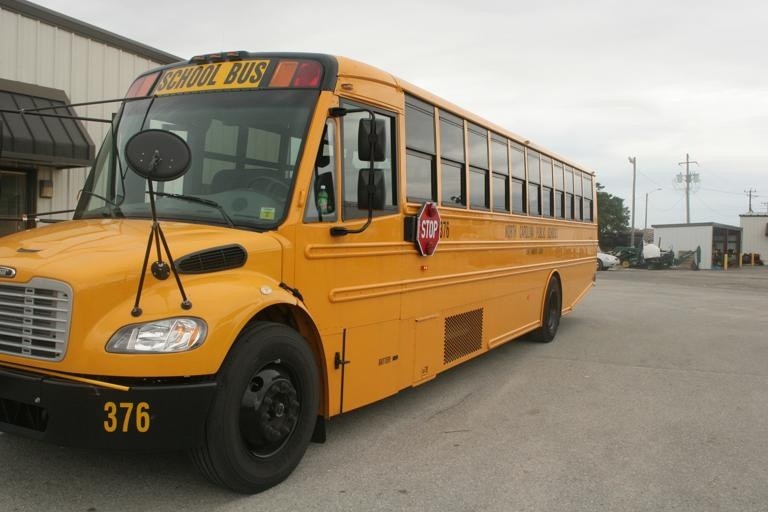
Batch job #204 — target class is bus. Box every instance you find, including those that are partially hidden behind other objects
[0,50,597,496]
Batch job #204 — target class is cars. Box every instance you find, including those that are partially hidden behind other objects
[596,245,620,270]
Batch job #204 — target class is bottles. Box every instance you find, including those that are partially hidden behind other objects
[316,184,329,215]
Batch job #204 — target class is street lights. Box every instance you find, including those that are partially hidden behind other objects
[627,155,637,250]
[644,187,663,241]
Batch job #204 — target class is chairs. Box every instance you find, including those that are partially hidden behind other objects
[211,168,284,193]
